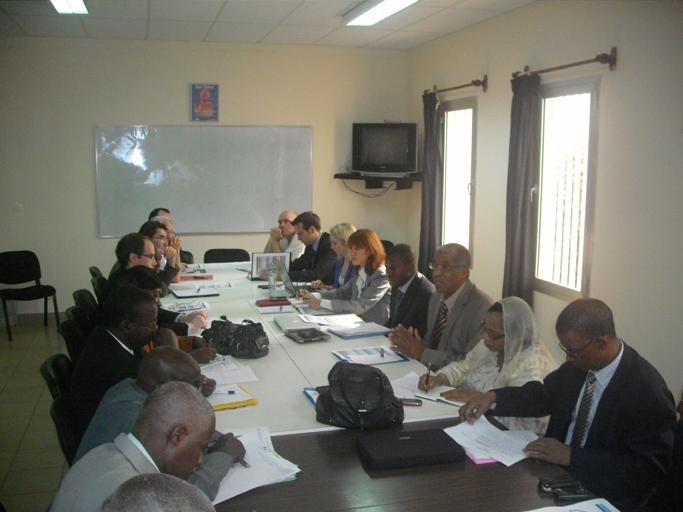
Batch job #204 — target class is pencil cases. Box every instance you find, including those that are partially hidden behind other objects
[212,390,237,399]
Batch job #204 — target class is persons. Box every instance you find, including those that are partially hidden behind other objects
[357,243,436,340]
[309,222,356,293]
[294,228,392,327]
[68,283,179,419]
[103,220,177,308]
[193,89,214,118]
[457,297,676,511]
[147,208,188,272]
[387,242,496,372]
[98,472,214,511]
[46,379,216,511]
[68,345,246,502]
[263,209,306,262]
[107,265,216,365]
[258,210,337,287]
[149,215,181,282]
[417,295,559,438]
[104,232,207,336]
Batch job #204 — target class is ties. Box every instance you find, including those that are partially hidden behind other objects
[569,372,596,449]
[431,302,449,351]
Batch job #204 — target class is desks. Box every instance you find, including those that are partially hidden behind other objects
[156,262,482,434]
[214,417,598,512]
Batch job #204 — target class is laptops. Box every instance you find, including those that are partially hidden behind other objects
[279,260,320,296]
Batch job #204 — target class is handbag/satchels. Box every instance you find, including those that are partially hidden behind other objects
[316,359,404,429]
[202,316,269,360]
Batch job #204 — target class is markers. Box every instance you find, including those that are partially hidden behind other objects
[380,349,383,358]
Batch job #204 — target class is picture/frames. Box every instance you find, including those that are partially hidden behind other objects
[190,83,220,123]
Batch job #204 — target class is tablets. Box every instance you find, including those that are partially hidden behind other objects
[285,328,330,343]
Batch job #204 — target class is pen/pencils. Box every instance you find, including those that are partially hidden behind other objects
[301,284,307,304]
[196,263,198,268]
[278,306,280,311]
[234,453,250,469]
[424,363,431,393]
[195,287,198,293]
[232,432,242,443]
[235,268,250,273]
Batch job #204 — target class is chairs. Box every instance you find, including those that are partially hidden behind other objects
[204,249,251,265]
[88,266,107,304]
[0,250,61,342]
[59,320,78,360]
[39,354,74,402]
[65,306,82,321]
[72,289,97,306]
[50,393,83,467]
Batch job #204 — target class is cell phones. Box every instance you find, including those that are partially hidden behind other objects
[402,397,421,407]
[553,482,590,502]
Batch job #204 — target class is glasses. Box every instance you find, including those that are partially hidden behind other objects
[479,319,504,340]
[557,340,595,356]
[143,254,156,259]
[153,234,167,240]
[427,262,468,271]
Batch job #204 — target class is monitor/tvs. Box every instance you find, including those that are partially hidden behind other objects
[349,118,419,178]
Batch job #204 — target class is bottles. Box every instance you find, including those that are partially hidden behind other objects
[267,268,276,298]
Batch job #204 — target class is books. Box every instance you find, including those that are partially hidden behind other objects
[204,383,260,412]
[255,299,291,306]
[286,297,310,309]
[464,448,499,464]
[213,423,299,485]
[250,251,291,280]
[167,280,220,299]
[413,384,466,407]
